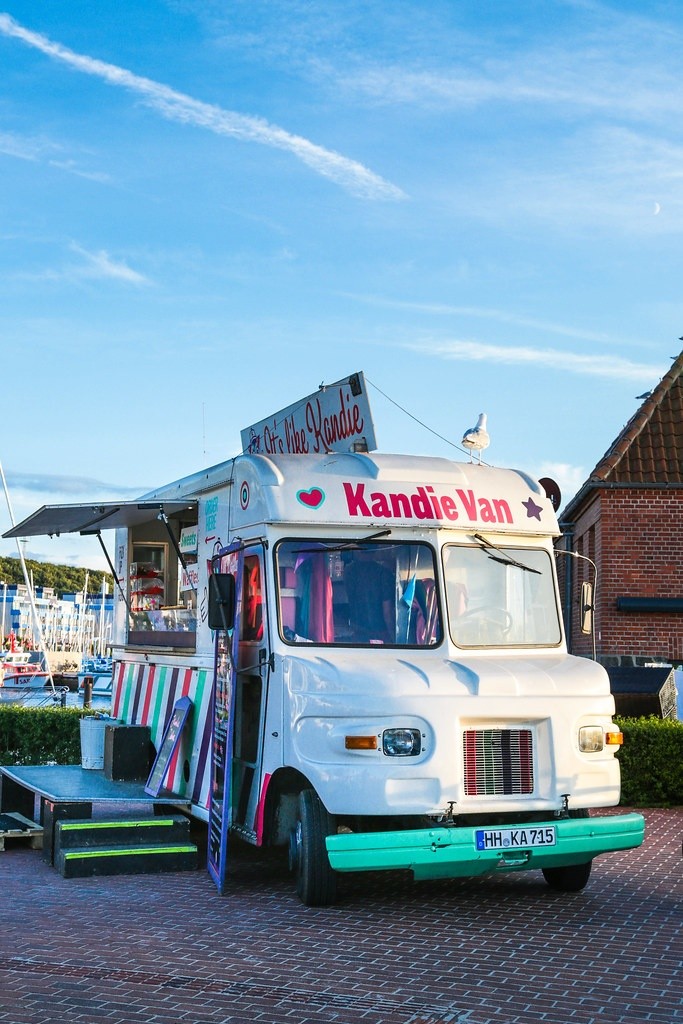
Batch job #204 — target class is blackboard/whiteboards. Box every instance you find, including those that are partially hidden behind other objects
[143,694,193,798]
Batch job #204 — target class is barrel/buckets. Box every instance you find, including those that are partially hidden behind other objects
[79,712,121,771]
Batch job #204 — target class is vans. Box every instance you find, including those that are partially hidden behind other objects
[0,435,645,908]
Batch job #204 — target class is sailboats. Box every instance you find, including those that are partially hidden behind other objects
[0,557,117,698]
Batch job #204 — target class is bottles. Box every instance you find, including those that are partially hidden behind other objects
[335,555,343,578]
[129,592,160,612]
[329,555,335,578]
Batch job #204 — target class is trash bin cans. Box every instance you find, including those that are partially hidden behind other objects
[79,718,124,770]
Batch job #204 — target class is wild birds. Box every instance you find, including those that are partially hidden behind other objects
[670,356,678,361]
[635,391,651,399]
[461,413,490,466]
